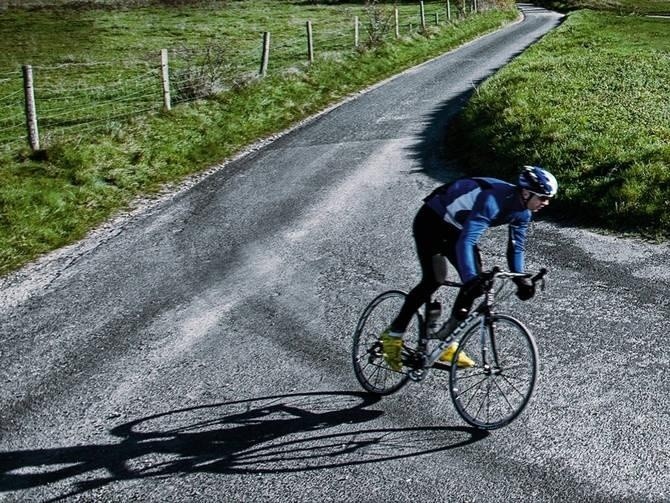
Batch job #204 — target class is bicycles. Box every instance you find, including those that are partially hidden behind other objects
[352,266,549,428]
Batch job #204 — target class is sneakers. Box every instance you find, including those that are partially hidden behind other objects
[379,329,403,371]
[439,342,475,368]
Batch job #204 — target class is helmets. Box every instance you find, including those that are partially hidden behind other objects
[518,164,560,198]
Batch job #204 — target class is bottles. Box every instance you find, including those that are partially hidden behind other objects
[428,297,442,334]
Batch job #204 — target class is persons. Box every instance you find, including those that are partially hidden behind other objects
[380,165,558,373]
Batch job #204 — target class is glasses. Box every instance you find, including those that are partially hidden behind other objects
[536,196,553,201]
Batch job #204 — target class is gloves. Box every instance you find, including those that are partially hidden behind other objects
[461,276,485,302]
[512,276,536,301]
